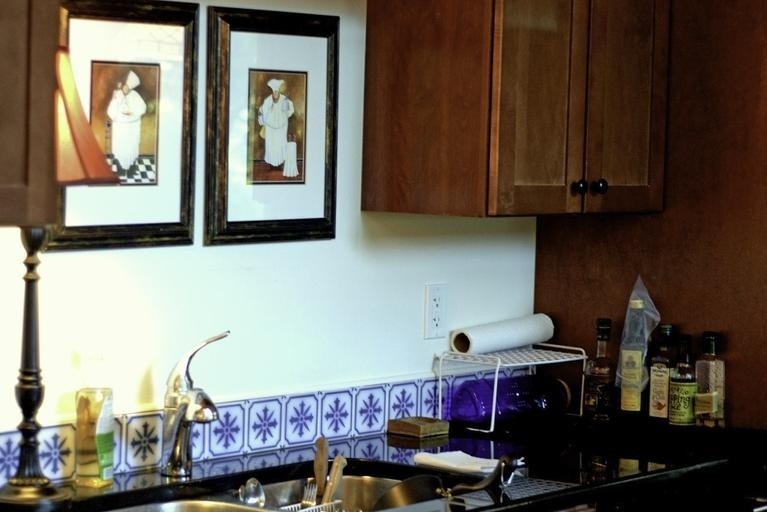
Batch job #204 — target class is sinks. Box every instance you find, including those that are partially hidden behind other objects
[231,473,404,511]
[110,500,268,512]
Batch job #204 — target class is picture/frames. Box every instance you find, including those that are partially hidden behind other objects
[205,5,336,245]
[48,1,199,246]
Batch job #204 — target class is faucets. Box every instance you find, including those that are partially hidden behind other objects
[160,330,231,477]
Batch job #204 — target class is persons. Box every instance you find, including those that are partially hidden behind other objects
[106,84,147,170]
[262,91,294,170]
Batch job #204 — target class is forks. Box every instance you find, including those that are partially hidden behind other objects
[302,484,317,507]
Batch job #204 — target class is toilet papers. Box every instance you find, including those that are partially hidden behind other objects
[449,313,554,355]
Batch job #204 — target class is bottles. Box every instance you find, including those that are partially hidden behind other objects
[451,377,571,424]
[585,299,725,425]
[75,389,115,487]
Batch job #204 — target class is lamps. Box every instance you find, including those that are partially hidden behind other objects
[0,9,120,508]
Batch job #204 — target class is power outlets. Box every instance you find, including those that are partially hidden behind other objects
[425,285,447,339]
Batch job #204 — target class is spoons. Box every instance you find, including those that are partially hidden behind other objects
[243,476,265,506]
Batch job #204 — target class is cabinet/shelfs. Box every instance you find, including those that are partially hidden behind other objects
[361,0,670,218]
[0,0,58,225]
